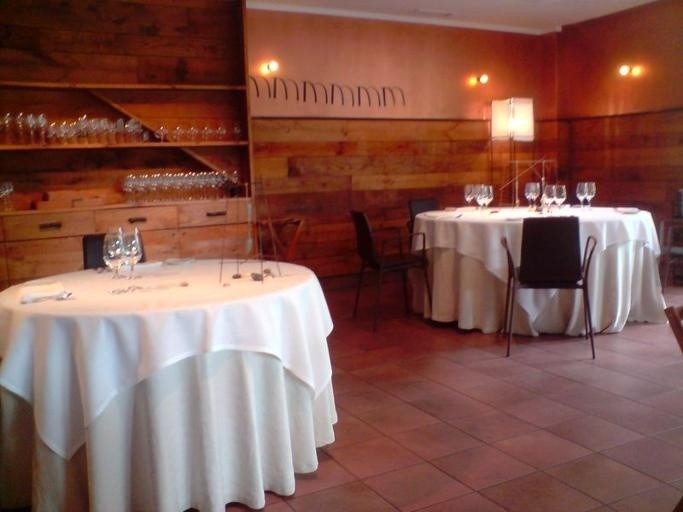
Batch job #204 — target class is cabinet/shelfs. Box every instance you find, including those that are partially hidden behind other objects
[0,1,259,290]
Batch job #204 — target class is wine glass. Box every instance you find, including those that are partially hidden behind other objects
[0,111,242,205]
[102,224,143,295]
[464,182,597,215]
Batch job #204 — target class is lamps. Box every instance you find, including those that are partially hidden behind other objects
[489,94,536,205]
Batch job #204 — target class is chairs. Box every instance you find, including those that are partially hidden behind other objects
[500,214,599,360]
[347,195,441,333]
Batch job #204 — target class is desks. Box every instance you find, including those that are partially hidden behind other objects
[1,259,319,512]
[412,205,653,335]
